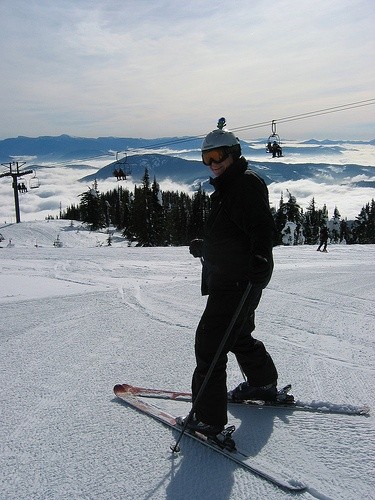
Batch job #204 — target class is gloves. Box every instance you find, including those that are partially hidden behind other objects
[189,239,204,258]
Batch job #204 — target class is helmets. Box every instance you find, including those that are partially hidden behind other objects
[202,129,240,151]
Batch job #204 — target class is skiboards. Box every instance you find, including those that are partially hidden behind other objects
[109,379,371,490]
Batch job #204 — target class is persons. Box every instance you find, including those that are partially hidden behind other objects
[266,141,284,158]
[175,129,280,436]
[113,168,127,181]
[17,182,29,194]
[316,222,330,252]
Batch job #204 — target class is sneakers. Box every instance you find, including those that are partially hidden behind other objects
[175,413,224,436]
[227,382,278,403]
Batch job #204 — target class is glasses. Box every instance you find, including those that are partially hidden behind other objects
[202,148,232,166]
